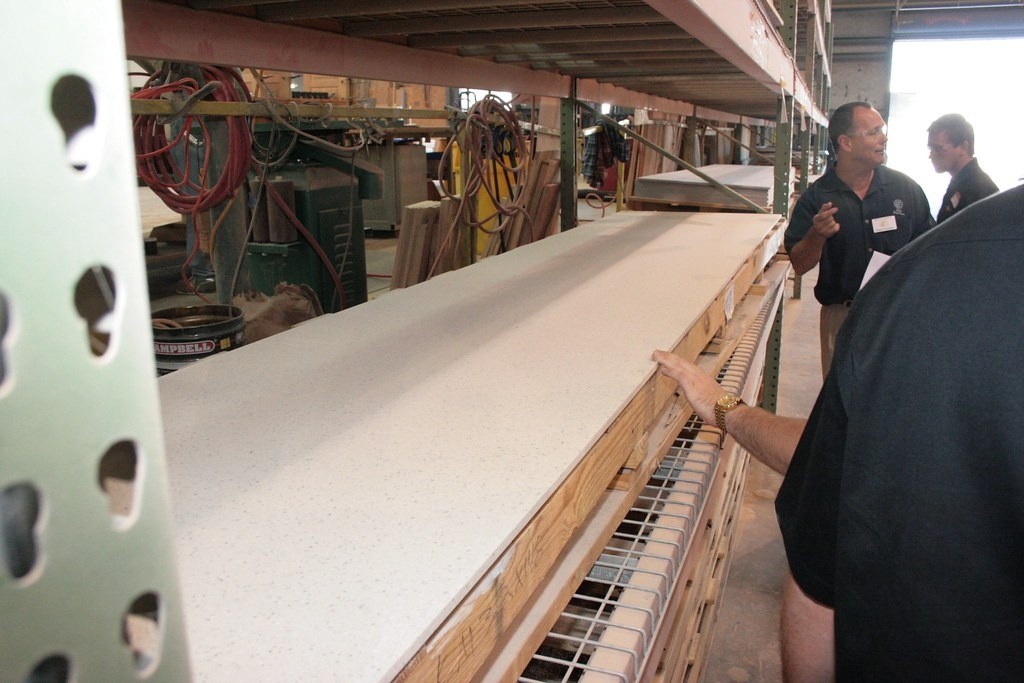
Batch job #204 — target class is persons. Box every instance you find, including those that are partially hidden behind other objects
[784,100,939,386]
[780,183,1023,683]
[653,349,810,477]
[927,113,1000,225]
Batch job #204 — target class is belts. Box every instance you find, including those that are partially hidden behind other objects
[838,299,853,307]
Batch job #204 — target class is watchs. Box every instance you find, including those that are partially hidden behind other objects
[715,393,749,435]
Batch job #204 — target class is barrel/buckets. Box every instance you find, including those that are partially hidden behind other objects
[150,303,245,376]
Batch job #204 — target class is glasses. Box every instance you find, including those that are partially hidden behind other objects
[848,126,888,138]
